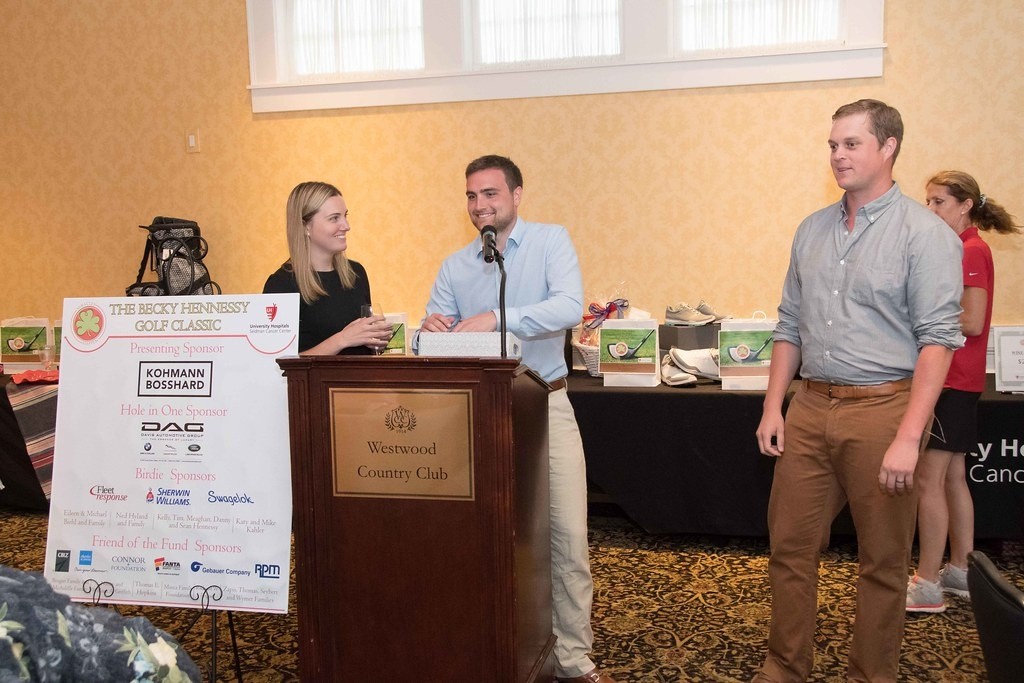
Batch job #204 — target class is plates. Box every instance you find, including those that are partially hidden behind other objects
[10,369,62,386]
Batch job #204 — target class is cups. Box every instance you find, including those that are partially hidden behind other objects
[38,345,55,370]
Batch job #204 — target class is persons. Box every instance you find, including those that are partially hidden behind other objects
[412,153,618,683]
[754,99,967,683]
[904,170,1024,613]
[262,181,392,356]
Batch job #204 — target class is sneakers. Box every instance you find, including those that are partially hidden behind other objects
[660,347,721,387]
[905,563,970,612]
[664,301,733,326]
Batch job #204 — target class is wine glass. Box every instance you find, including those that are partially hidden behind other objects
[360,303,387,356]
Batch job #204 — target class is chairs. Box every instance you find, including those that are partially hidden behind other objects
[967,550,1024,682]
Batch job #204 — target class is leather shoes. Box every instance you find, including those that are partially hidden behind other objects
[553,666,616,683]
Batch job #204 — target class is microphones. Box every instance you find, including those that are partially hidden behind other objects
[481,224,498,263]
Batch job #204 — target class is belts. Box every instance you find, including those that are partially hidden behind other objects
[800,377,911,399]
[548,376,568,392]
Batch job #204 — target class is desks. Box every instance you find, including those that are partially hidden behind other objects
[566,370,1023,561]
